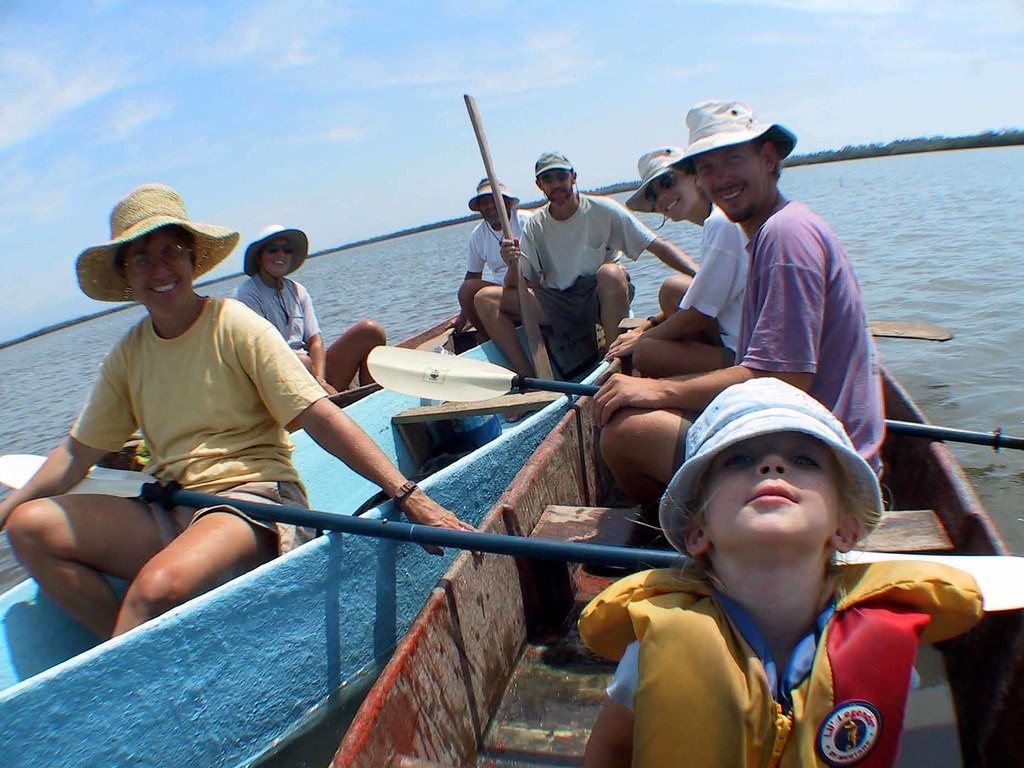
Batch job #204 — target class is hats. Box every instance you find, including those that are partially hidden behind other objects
[244,225,308,279]
[535,152,573,178]
[625,146,685,213]
[76,183,240,301]
[469,178,520,211]
[666,97,797,175]
[658,377,886,560]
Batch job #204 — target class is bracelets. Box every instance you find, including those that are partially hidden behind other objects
[394,481,417,510]
[647,316,658,327]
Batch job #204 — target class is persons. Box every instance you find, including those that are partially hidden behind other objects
[593,100,886,548]
[447,178,533,343]
[233,225,387,396]
[577,376,983,768]
[0,185,481,639]
[604,147,750,378]
[474,152,701,423]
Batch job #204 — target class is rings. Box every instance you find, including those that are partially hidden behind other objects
[460,526,464,531]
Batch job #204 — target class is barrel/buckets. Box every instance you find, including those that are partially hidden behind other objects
[427,411,501,454]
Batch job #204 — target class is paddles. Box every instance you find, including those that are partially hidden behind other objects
[463,92,556,382]
[616,315,957,342]
[0,450,1024,618]
[365,343,998,448]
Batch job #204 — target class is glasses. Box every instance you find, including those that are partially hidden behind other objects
[122,241,194,275]
[537,171,573,183]
[645,168,692,202]
[261,243,295,253]
